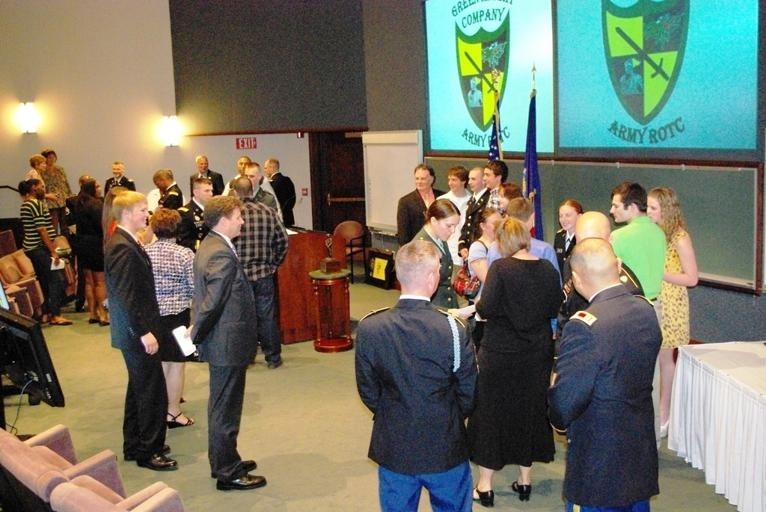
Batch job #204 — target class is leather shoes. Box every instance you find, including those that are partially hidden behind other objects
[99,320,110,326]
[136,451,178,471]
[210,458,257,478]
[512,480,532,501]
[472,486,495,508]
[89,318,99,323]
[217,473,267,491]
[267,359,283,369]
[123,443,171,461]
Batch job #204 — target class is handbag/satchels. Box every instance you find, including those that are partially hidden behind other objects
[451,267,481,298]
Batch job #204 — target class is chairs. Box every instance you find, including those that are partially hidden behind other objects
[332,218,368,284]
[0,247,45,318]
[1,422,186,512]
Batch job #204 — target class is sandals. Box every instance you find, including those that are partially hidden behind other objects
[166,413,194,428]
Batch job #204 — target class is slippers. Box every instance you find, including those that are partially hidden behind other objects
[50,317,71,326]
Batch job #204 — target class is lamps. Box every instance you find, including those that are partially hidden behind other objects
[162,116,179,149]
[20,102,37,135]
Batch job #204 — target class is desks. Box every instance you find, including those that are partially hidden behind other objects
[665,338,764,512]
[305,268,354,352]
[272,222,349,346]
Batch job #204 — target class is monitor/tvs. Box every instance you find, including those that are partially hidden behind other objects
[0,307,65,408]
